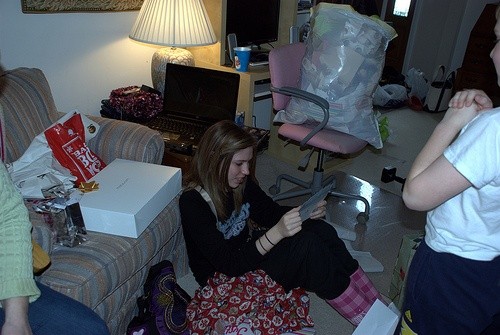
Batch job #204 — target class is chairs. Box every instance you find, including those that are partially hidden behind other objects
[269,43,370,224]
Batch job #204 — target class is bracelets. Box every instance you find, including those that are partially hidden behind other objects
[265,233,276,247]
[259,237,268,253]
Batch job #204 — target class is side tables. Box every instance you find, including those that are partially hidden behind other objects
[153,120,270,182]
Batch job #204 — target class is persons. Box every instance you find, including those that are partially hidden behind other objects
[0,160,108,335]
[402,8,500,335]
[179,120,402,335]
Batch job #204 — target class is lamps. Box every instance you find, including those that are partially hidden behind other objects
[129,0,217,98]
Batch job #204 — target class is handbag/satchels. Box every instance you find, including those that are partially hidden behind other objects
[405,67,429,99]
[390,232,424,312]
[424,63,456,113]
[43,114,107,187]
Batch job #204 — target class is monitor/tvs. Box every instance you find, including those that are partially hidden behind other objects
[226,0,280,53]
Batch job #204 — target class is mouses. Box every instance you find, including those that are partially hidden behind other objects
[174,140,193,151]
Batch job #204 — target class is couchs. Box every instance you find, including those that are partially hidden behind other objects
[0,67,189,335]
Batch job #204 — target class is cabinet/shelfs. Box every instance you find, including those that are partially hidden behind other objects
[188,0,297,127]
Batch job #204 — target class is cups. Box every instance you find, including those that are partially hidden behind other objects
[233,46,252,72]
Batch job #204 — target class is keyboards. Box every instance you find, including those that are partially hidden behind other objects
[249,51,271,62]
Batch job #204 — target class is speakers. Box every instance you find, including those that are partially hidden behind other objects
[227,33,238,67]
[290,25,299,44]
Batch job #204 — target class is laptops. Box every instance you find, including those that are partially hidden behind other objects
[143,63,239,149]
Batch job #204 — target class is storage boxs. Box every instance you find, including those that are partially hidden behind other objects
[74,158,183,239]
